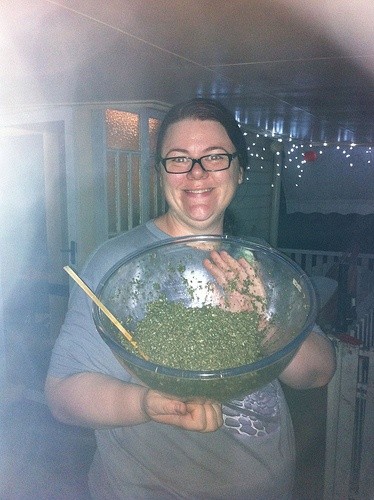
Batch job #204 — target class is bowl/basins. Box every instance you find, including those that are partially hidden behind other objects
[95,235,317,399]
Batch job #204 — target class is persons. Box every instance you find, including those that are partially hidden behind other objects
[45,98,337,500]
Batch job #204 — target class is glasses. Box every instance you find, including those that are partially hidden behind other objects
[160,152,237,174]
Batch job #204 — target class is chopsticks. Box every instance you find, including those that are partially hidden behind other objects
[62,264,148,364]
